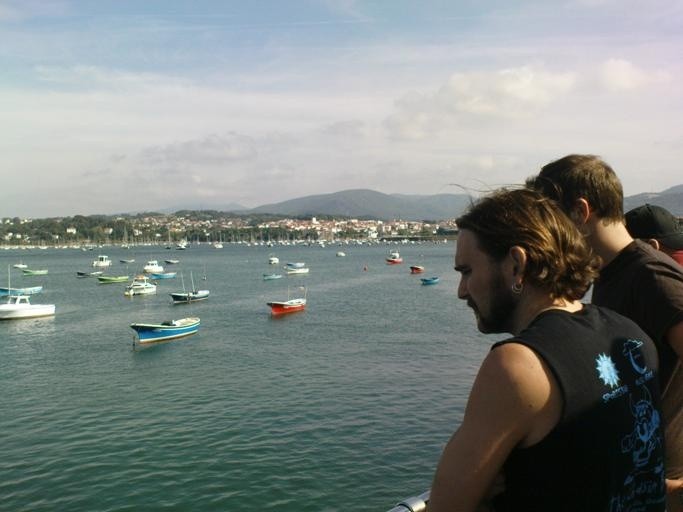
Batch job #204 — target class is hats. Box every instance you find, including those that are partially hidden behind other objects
[624,202,683,251]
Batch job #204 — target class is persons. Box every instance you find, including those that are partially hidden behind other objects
[524,153,683,511]
[418,183,666,511]
[621,201,683,266]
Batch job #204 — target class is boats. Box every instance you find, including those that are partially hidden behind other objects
[13,263,27,268]
[409,265,426,275]
[23,269,49,276]
[0,296,56,320]
[264,286,309,316]
[74,254,179,296]
[419,276,441,286]
[130,316,200,344]
[385,250,401,264]
[260,255,309,282]
[0,286,42,296]
[169,270,210,305]
[335,251,345,257]
